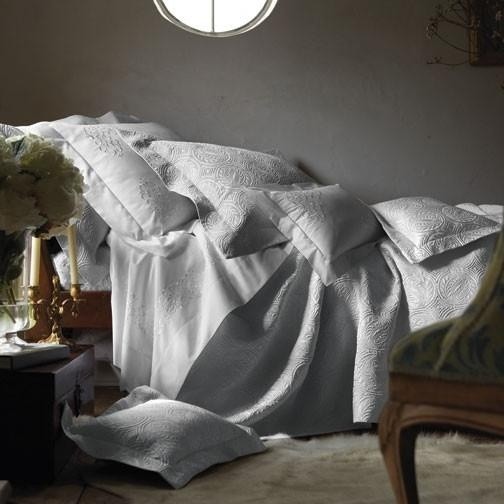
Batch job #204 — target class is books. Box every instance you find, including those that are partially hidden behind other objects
[0,344,70,369]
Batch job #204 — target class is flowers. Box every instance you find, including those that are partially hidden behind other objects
[2,119,85,322]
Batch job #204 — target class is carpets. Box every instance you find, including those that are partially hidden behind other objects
[86,432,504,504]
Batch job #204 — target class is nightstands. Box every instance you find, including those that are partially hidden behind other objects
[0,343,96,495]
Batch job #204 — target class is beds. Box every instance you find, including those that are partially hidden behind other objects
[29,203,504,437]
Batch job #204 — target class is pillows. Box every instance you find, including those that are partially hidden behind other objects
[115,127,319,260]
[14,111,199,272]
[369,197,503,264]
[61,386,272,489]
[225,183,388,287]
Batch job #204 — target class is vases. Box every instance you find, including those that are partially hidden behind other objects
[0,300,37,358]
